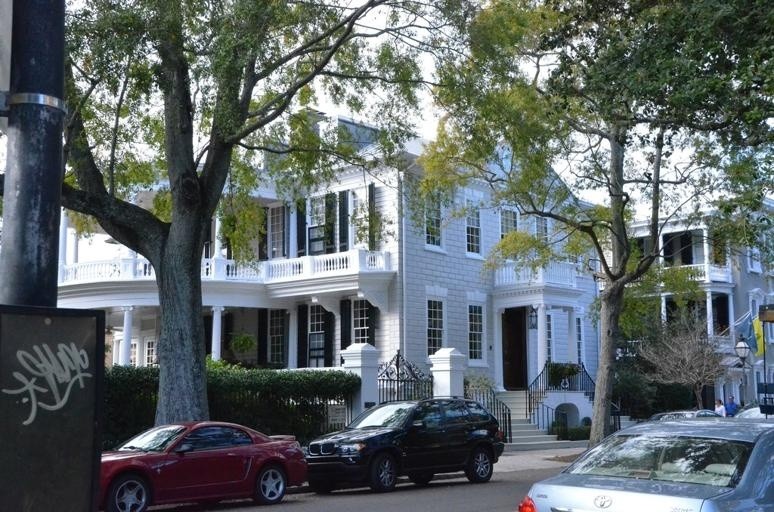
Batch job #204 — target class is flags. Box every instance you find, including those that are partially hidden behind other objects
[731,312,758,356]
[749,316,767,357]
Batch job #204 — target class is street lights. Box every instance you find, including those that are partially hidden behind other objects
[734,334,751,408]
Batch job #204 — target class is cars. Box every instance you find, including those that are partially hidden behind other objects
[306,396,505,495]
[99,421,308,510]
[645,409,723,421]
[518,416,774,512]
[734,406,774,420]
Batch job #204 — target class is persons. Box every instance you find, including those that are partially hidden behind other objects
[714,399,725,418]
[724,395,737,416]
